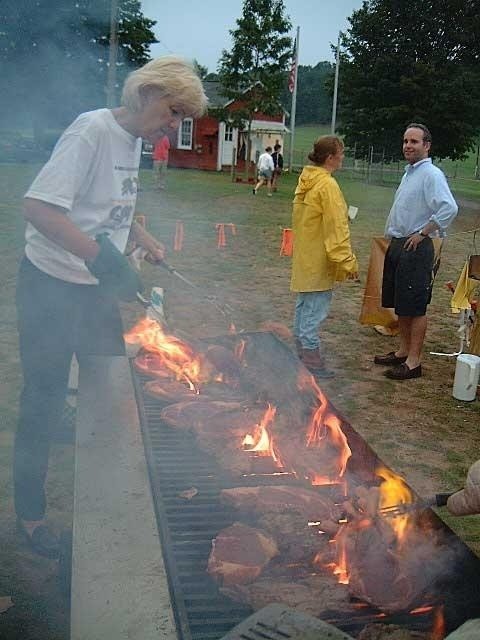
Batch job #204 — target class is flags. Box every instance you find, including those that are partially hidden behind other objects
[288,36,296,94]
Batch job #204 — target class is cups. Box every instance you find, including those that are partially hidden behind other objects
[346,205,359,221]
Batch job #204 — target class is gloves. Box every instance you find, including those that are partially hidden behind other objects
[85,230,146,302]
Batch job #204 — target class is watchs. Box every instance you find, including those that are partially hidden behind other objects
[419,230,429,237]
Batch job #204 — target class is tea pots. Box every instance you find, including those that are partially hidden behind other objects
[452,353,480,403]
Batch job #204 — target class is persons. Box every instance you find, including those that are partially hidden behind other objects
[271,145,283,192]
[15,59,210,556]
[253,146,274,197]
[373,123,458,380]
[151,134,170,191]
[287,136,365,381]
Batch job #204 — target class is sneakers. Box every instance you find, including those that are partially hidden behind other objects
[253,188,257,195]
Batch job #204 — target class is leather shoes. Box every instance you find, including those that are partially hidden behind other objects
[382,361,422,381]
[14,512,63,560]
[310,367,336,379]
[374,351,409,367]
